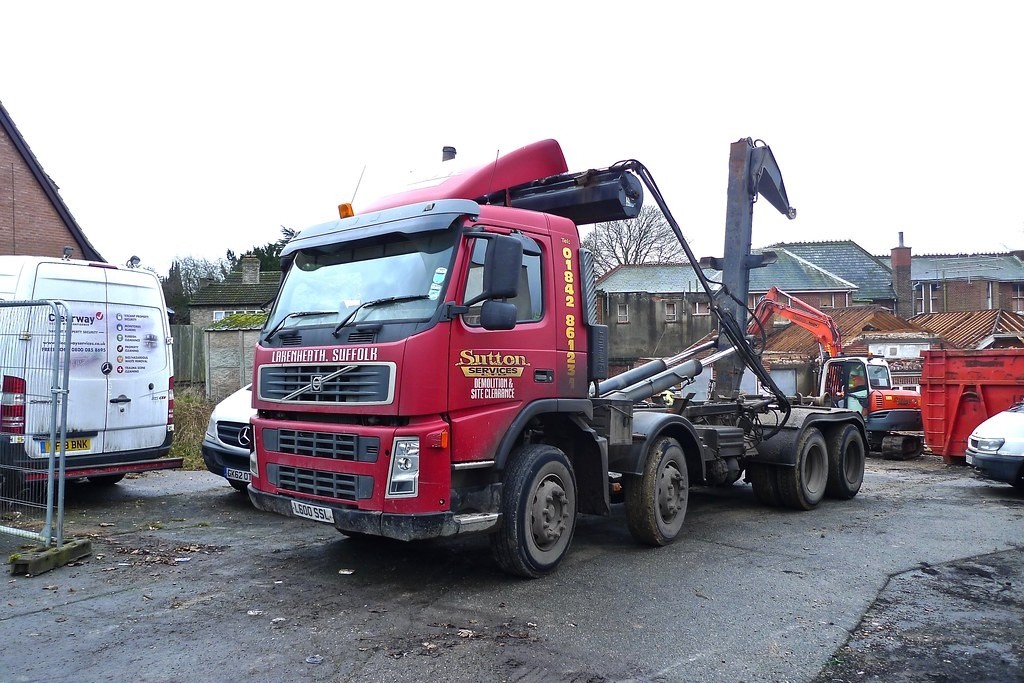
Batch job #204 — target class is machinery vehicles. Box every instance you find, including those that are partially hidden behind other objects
[741,283,924,457]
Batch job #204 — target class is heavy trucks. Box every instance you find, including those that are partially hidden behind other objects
[256,137,870,583]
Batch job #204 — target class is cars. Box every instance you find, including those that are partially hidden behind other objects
[200,382,254,494]
[963,400,1024,489]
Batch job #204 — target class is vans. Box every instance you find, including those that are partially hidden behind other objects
[1,245,185,501]
